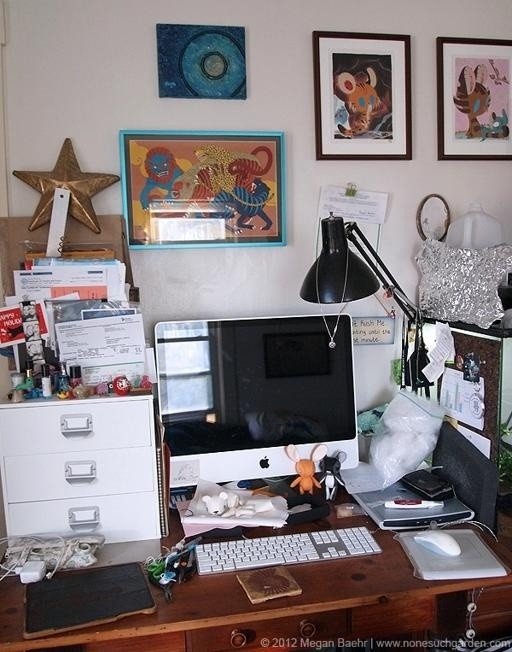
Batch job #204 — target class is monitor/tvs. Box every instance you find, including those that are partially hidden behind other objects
[154,313,359,497]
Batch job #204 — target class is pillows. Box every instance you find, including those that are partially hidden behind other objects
[243,409,331,443]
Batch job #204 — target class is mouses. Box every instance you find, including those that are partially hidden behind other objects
[413,529,462,557]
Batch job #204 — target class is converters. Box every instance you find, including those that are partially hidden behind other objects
[19,560,47,584]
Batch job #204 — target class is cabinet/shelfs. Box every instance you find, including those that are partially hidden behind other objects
[0,388,163,554]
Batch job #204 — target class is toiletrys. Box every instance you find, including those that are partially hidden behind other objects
[10,356,89,403]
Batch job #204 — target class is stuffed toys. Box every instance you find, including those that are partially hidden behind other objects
[200,491,256,518]
[317,450,347,501]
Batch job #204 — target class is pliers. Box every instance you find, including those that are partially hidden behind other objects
[150,575,175,604]
[171,550,194,584]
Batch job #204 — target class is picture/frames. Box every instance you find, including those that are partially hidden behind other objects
[312,30,413,163]
[116,127,288,251]
[436,36,512,162]
[263,332,333,380]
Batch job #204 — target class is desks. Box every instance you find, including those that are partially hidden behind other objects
[0,480,511,652]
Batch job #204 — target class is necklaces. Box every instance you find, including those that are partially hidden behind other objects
[314,242,351,350]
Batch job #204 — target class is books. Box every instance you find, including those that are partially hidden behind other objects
[235,565,303,606]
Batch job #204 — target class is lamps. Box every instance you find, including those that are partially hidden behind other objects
[301,210,434,401]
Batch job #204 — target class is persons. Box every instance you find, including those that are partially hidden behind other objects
[19,303,45,356]
[284,443,329,497]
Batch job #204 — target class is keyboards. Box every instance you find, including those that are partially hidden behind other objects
[194,526,384,576]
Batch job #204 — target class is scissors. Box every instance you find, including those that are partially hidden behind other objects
[147,561,165,579]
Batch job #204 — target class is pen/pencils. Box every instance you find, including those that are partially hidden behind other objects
[165,536,202,565]
